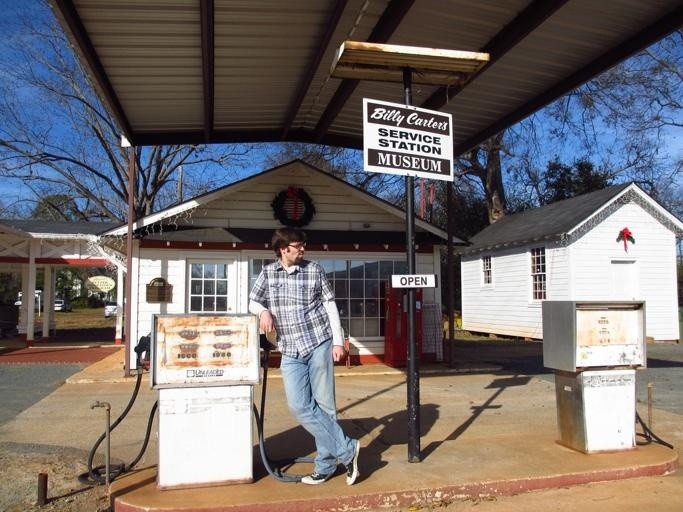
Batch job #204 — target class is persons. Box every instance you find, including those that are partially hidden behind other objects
[248,226,360,486]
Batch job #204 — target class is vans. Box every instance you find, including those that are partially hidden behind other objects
[53,299,72,312]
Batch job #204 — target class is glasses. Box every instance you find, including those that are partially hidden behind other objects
[288,241,307,249]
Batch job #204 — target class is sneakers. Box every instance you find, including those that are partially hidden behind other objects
[346,440,360,486]
[301,470,337,485]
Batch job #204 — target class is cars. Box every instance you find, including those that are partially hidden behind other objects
[105,301,118,318]
